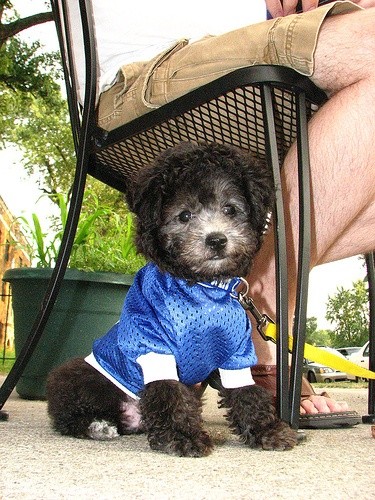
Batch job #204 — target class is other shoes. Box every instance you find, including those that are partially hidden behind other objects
[251,365,363,427]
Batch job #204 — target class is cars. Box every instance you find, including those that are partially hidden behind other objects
[349,340,369,384]
[303,344,350,384]
[337,346,362,360]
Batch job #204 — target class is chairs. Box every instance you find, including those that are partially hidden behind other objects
[0,0,375,435]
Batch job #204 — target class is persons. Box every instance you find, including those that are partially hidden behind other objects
[59,0,375,427]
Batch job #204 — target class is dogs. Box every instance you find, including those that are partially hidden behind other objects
[42,140,302,459]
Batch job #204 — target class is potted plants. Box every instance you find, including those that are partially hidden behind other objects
[2,188,149,401]
